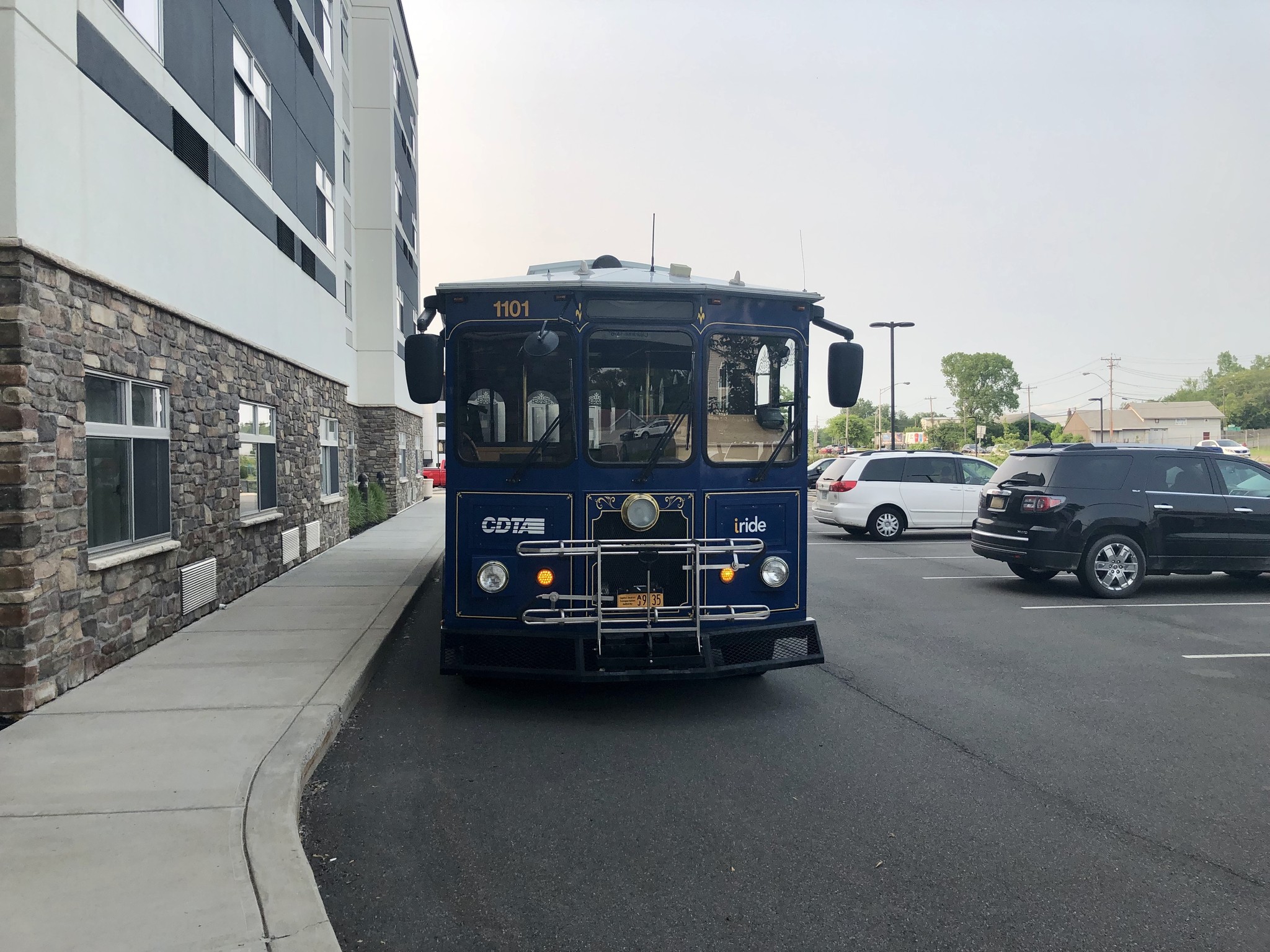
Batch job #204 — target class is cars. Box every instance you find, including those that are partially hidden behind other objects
[818,444,846,455]
[986,446,994,453]
[807,457,837,491]
[844,448,857,454]
[1196,439,1251,458]
[931,447,942,450]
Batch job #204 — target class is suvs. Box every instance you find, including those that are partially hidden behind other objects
[811,449,999,542]
[961,444,987,454]
[992,444,1017,455]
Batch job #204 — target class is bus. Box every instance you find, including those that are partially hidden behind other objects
[404,255,864,683]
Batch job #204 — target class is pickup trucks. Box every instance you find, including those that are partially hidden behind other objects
[423,459,446,488]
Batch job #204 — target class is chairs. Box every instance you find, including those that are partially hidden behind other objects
[940,466,951,483]
[1146,464,1211,494]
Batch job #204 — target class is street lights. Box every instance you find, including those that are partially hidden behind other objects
[946,406,966,442]
[879,382,910,451]
[1082,372,1113,443]
[966,415,977,457]
[1088,397,1103,443]
[869,321,915,450]
